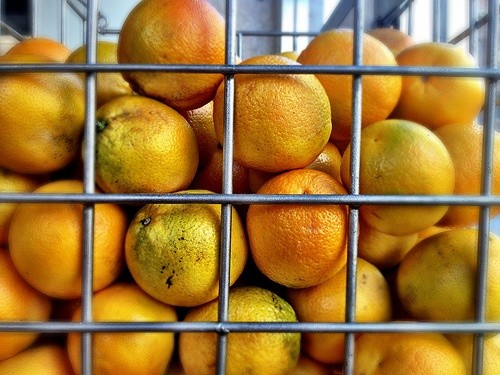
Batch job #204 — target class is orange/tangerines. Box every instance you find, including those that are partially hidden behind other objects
[0,29,500,375]
[117,0,227,110]
